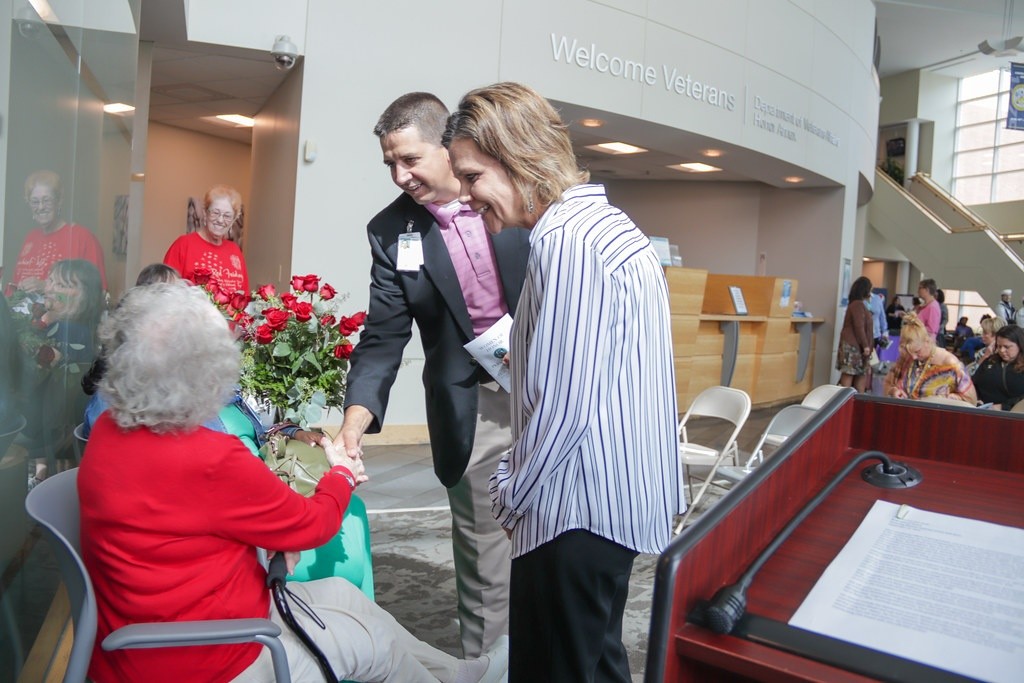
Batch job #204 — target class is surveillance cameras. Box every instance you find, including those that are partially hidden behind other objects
[272,36,298,69]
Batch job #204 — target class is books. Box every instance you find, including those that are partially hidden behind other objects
[977,402,993,410]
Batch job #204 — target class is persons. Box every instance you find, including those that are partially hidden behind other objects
[10,169,109,301]
[84,264,376,605]
[830,274,1023,414]
[441,81,686,683]
[75,280,510,683]
[1,260,107,460]
[332,92,535,661]
[838,276,874,394]
[165,186,250,330]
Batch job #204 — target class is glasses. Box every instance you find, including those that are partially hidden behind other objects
[28,196,56,207]
[207,208,236,221]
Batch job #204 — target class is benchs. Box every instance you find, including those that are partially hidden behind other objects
[943,330,974,358]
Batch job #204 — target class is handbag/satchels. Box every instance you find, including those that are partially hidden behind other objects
[257,421,333,498]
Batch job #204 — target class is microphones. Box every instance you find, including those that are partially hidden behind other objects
[702,451,895,635]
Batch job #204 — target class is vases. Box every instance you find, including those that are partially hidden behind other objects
[244,394,277,429]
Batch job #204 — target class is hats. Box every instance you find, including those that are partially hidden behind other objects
[1001,289,1012,295]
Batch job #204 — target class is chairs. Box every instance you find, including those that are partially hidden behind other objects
[674,384,856,535]
[24,466,292,683]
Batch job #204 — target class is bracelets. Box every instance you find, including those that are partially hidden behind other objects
[333,471,355,489]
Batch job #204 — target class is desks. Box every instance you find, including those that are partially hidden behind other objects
[662,266,826,421]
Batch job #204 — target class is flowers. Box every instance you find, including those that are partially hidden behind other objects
[194,264,368,429]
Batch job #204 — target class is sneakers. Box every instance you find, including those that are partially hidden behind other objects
[476,634,509,683]
